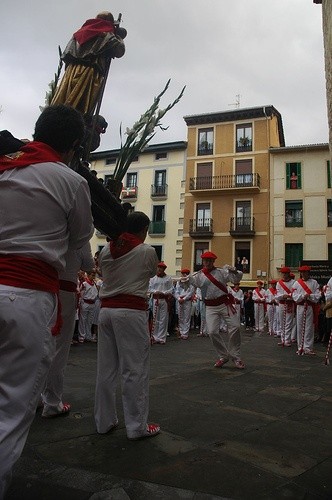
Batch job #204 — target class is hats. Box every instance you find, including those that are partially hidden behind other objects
[257,281,263,285]
[280,267,291,272]
[271,280,277,284]
[158,262,167,268]
[181,268,190,273]
[201,251,217,259]
[298,265,311,271]
[289,273,296,277]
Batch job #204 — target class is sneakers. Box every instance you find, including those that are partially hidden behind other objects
[232,359,247,369]
[214,358,229,367]
[127,423,160,440]
[41,402,72,418]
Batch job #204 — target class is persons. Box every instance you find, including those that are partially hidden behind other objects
[41,241,94,419]
[0,107,95,500]
[187,252,246,370]
[290,172,298,189]
[227,267,332,356]
[71,262,210,344]
[95,208,160,441]
[236,256,248,273]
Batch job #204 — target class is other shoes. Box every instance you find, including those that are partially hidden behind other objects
[291,338,296,342]
[285,340,291,346]
[79,334,84,342]
[153,328,281,343]
[296,348,303,355]
[277,340,285,345]
[304,351,317,355]
